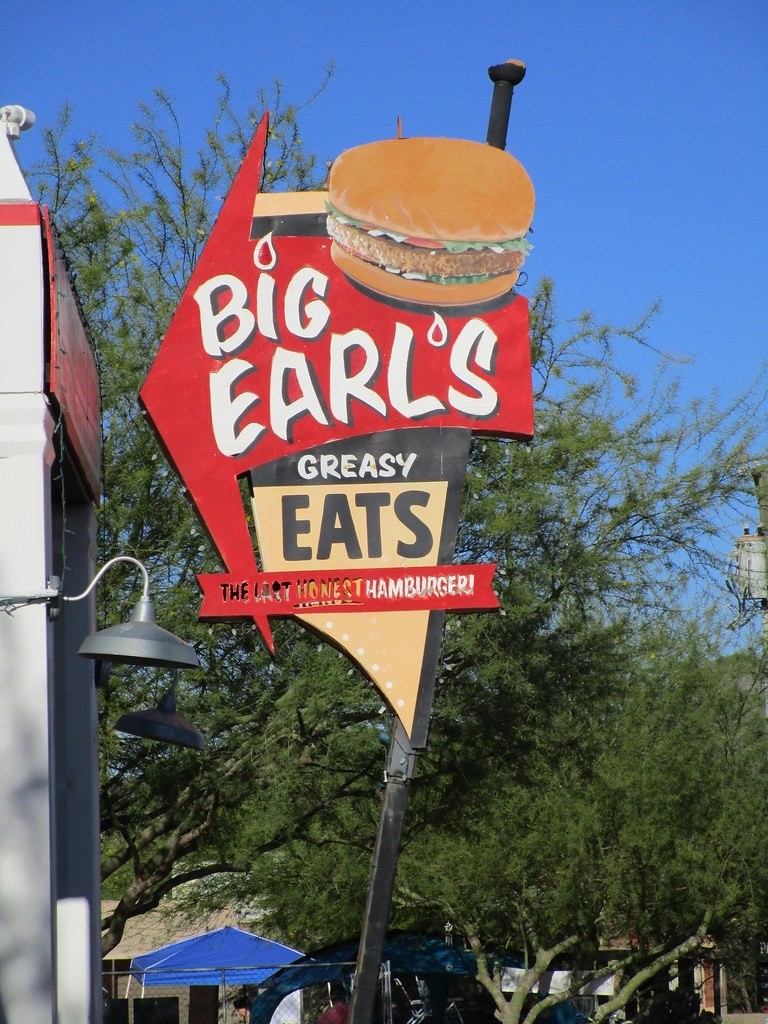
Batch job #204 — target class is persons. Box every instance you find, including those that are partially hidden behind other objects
[315,983,348,1024]
[232,995,251,1024]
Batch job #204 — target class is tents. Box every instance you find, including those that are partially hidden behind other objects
[243,927,577,1023]
[121,925,335,1024]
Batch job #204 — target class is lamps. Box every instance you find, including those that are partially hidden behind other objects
[95,660,206,751]
[0,555,200,669]
